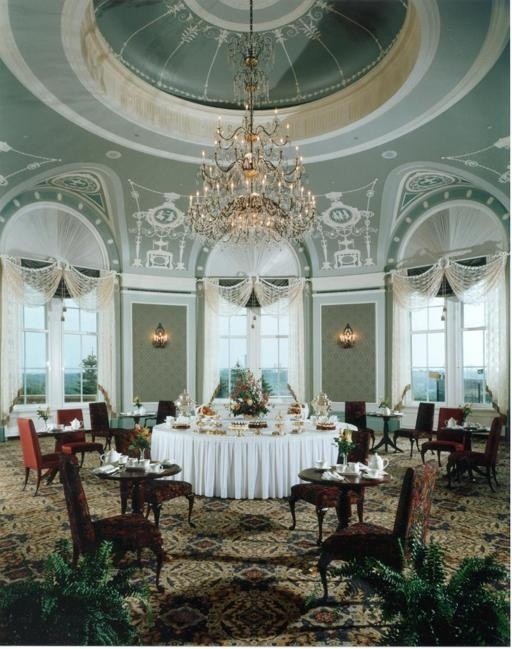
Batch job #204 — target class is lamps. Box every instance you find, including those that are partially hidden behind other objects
[151,324,168,348]
[338,324,357,350]
[182,0,319,251]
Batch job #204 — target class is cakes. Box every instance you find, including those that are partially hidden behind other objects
[317,420,336,430]
[249,422,267,428]
[231,422,249,428]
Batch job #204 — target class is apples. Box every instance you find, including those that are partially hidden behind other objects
[200,406,215,415]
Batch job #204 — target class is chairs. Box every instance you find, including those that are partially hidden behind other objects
[88,402,126,450]
[344,400,374,449]
[17,418,65,496]
[62,465,165,593]
[288,429,370,546]
[394,403,435,459]
[143,400,176,427]
[445,417,505,493]
[421,408,468,466]
[115,430,197,531]
[318,460,439,603]
[55,409,105,468]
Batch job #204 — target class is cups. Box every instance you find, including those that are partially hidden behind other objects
[369,407,400,416]
[34,416,84,433]
[93,446,176,477]
[313,452,391,482]
[165,396,342,435]
[444,417,491,433]
